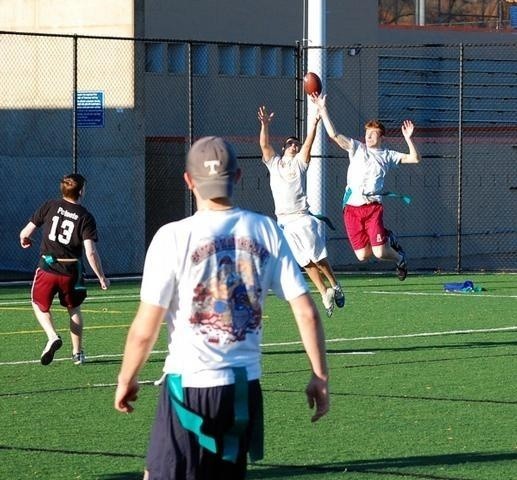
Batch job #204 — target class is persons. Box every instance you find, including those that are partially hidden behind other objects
[18,172,111,367]
[113,133,332,480]
[256,104,346,320]
[308,91,422,280]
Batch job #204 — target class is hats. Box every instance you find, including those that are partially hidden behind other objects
[186,136,237,200]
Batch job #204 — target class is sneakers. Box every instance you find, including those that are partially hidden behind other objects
[40,336,62,365]
[322,288,335,318]
[385,228,405,255]
[395,255,407,281]
[71,350,83,364]
[334,283,345,308]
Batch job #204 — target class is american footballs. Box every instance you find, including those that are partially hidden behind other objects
[303,72,321,98]
